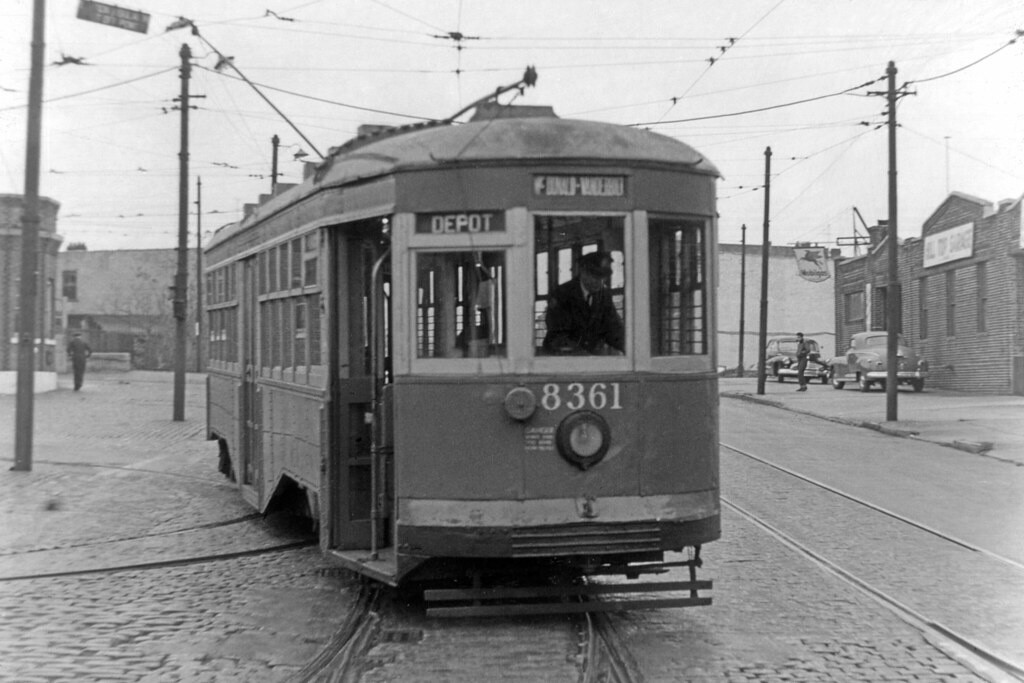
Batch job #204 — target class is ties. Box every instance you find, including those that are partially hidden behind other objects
[587,294,591,304]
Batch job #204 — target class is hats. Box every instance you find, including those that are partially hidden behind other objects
[578,251,612,277]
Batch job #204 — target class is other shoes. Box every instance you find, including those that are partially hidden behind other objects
[797,387,807,391]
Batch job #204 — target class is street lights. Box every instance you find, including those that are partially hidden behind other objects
[174,44,235,422]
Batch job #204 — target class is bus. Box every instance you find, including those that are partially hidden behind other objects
[204,104,722,619]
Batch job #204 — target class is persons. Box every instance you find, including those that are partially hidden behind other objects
[544,252,625,354]
[67,333,91,389]
[796,332,810,390]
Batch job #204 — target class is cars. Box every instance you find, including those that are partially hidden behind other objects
[832,331,931,392]
[766,336,831,383]
